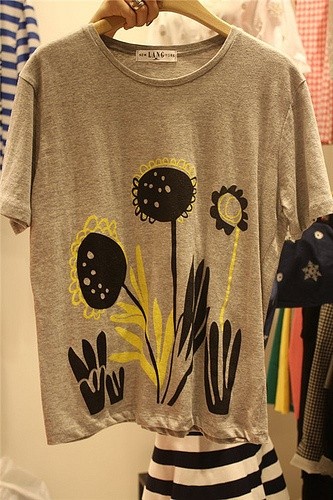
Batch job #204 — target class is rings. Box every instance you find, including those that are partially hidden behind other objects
[132,0,145,10]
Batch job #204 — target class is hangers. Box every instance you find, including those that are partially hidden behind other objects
[94,0,232,38]
